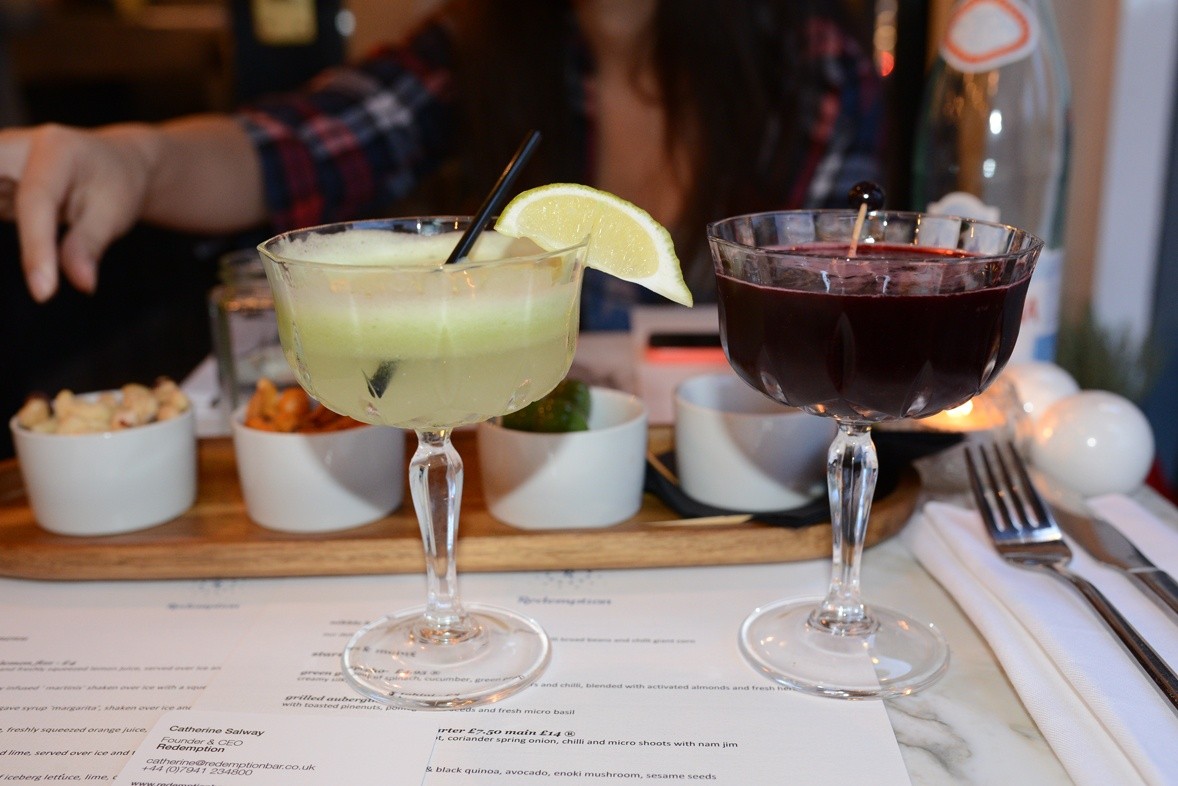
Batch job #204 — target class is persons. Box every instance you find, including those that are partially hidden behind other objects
[0,0,916,337]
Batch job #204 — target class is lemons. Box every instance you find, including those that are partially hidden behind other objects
[494,181,697,307]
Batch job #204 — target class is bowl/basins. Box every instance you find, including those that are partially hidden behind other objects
[231,396,405,533]
[8,389,198,535]
[477,387,646,529]
[674,374,840,511]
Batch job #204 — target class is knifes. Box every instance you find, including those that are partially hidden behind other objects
[1041,500,1178,619]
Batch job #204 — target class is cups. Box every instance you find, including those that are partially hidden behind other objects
[209,248,308,412]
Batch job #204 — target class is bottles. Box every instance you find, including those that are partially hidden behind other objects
[913,1,1072,370]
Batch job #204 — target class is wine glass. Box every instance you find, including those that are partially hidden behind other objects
[707,209,1045,700]
[256,215,590,702]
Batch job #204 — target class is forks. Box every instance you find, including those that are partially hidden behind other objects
[963,440,1178,711]
